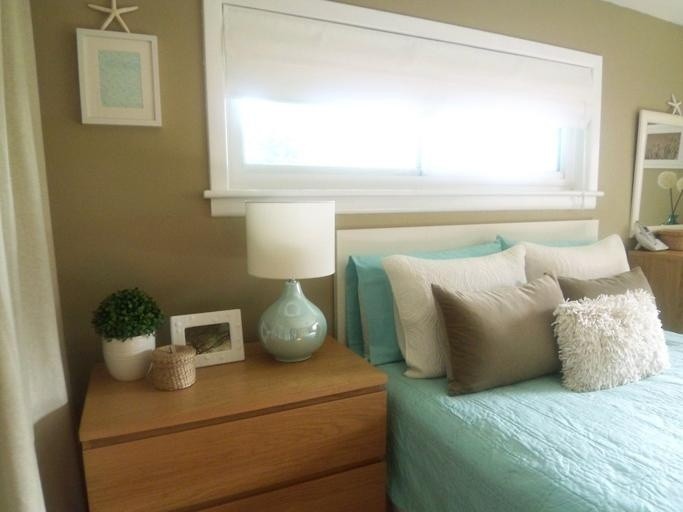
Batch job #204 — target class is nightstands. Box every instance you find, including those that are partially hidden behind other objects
[75,334,385,512]
[626,247,683,335]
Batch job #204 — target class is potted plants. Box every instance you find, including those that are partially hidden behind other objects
[93,286,162,386]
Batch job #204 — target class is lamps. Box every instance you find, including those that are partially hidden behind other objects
[242,199,339,363]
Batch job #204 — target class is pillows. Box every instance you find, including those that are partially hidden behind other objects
[548,287,673,395]
[525,229,632,282]
[496,233,591,254]
[429,270,568,397]
[344,240,504,369]
[557,263,655,302]
[379,241,530,378]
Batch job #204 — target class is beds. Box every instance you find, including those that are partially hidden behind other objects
[336,217,683,512]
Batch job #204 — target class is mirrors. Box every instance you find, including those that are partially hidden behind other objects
[628,110,683,238]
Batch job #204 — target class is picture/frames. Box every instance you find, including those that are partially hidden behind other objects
[170,308,244,369]
[73,26,165,132]
[643,124,682,170]
[203,0,607,219]
